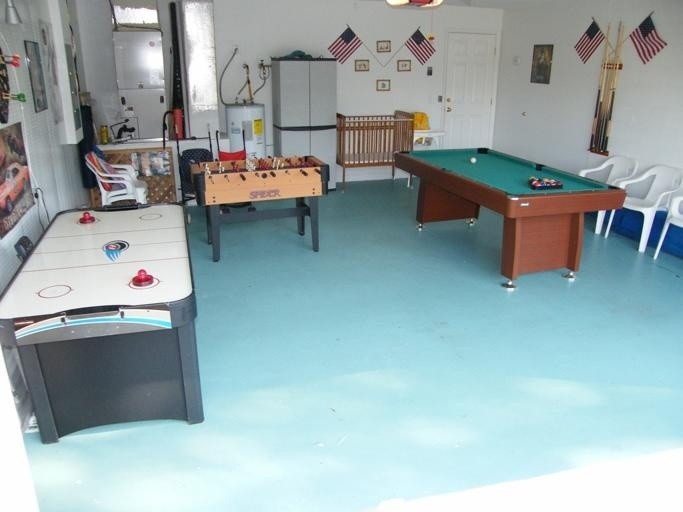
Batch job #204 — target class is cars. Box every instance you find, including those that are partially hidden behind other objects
[1,162,29,215]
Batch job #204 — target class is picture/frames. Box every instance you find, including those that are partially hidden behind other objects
[24,40,49,113]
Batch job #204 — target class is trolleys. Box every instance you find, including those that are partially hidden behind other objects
[216,129,256,214]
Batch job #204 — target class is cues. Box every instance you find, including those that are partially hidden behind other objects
[588,20,624,152]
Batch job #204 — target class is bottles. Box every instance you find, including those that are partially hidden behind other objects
[100,125,108,144]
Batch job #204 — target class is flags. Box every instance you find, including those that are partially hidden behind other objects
[626,14,669,66]
[571,20,606,66]
[326,26,364,66]
[404,28,436,66]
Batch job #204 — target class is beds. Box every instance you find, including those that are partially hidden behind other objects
[335,110,415,194]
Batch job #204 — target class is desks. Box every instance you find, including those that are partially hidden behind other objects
[392,148,627,281]
[0,204,206,444]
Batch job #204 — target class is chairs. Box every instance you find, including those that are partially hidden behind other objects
[577,155,683,260]
[85,151,149,207]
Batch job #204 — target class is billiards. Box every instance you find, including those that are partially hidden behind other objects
[471,157,476,163]
[528,176,562,189]
